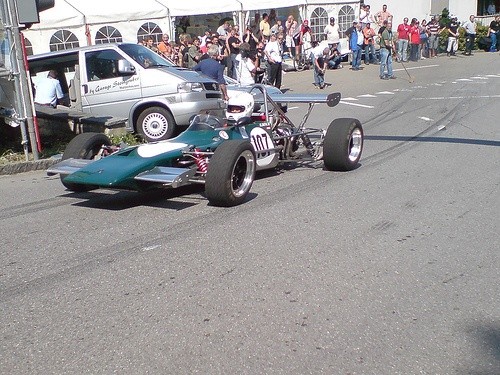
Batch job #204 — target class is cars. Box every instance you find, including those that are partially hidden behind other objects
[221,73,288,120]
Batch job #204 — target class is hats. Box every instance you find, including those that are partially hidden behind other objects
[330,17,334,21]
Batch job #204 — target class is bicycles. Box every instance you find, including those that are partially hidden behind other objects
[291,41,316,71]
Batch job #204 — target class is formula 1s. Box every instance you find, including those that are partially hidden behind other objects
[47,82,365,211]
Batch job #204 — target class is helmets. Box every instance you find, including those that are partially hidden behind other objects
[226,93,254,126]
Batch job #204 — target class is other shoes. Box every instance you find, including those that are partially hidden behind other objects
[381,75,389,80]
[352,67,363,70]
[452,52,456,56]
[388,74,397,79]
[447,52,450,56]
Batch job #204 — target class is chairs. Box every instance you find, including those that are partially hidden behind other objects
[249,83,268,123]
[68,64,100,105]
[102,59,115,79]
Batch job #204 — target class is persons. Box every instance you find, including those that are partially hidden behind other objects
[298,20,311,46]
[136,9,287,101]
[345,4,396,80]
[34,70,63,107]
[285,15,298,59]
[313,44,343,89]
[461,15,477,56]
[447,16,459,57]
[396,15,446,63]
[487,1,496,15]
[223,93,254,123]
[324,16,340,47]
[487,15,500,52]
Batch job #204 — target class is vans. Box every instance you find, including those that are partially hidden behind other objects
[14,42,229,144]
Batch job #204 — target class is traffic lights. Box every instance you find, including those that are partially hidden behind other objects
[14,0,55,26]
